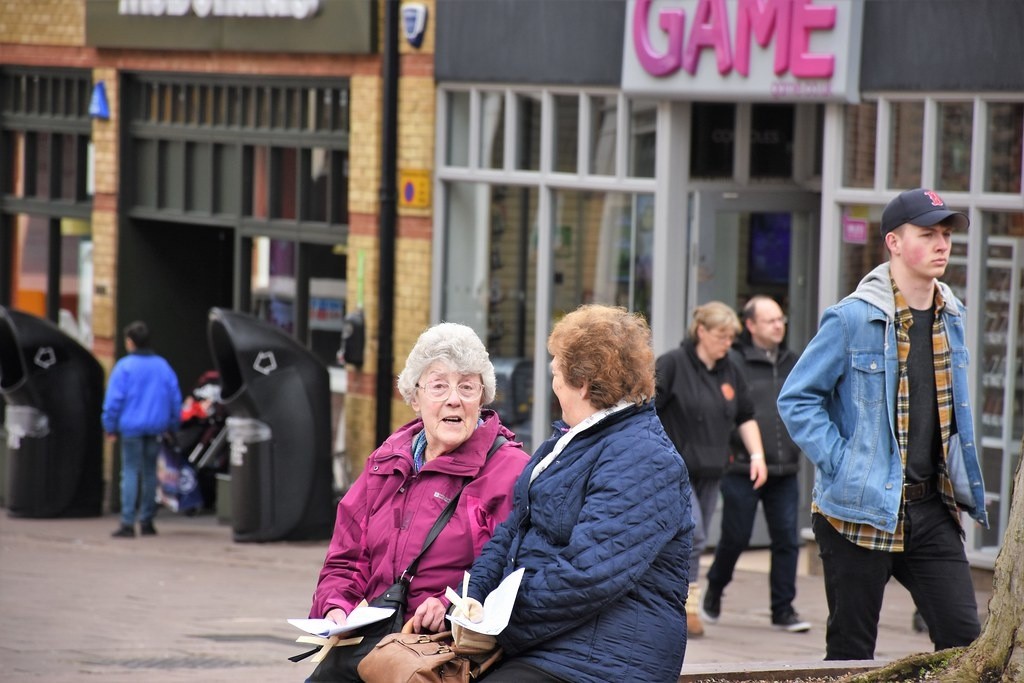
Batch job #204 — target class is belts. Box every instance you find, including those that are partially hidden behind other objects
[901,480,938,505]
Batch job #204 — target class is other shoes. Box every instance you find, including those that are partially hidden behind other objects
[110,525,136,536]
[140,521,157,535]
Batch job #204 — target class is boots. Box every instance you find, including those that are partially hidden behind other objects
[683,580,705,638]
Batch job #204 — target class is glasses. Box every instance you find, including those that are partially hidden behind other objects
[752,314,789,326]
[415,378,486,402]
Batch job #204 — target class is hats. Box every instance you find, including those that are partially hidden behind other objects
[880,188,970,232]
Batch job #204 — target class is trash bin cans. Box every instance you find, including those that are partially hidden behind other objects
[1,306,106,521]
[207,306,337,544]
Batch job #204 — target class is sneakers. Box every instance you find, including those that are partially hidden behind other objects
[698,577,723,623]
[769,603,813,633]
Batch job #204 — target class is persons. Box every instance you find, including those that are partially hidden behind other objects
[305,322,531,683]
[443,304,695,683]
[777,187,991,662]
[701,296,812,635]
[99,322,182,538]
[653,301,766,638]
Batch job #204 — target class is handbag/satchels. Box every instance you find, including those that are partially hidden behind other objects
[357,617,506,683]
[288,578,411,683]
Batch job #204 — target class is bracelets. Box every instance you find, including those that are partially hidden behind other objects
[750,453,765,460]
